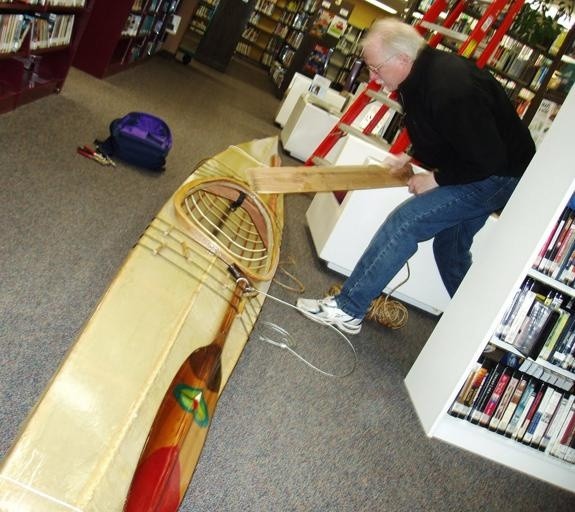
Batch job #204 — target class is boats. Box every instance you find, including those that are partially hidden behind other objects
[0,135,284,512]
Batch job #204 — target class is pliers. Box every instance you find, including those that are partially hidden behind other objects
[79,144,108,166]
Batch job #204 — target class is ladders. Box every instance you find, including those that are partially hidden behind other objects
[301,0,526,204]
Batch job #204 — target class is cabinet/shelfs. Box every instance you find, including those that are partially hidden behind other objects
[1,0,274,113]
[274,1,574,100]
[403,87,574,493]
[307,126,504,316]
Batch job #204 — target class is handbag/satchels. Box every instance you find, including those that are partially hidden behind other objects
[102,111,172,171]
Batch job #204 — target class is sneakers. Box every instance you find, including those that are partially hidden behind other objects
[295,295,362,335]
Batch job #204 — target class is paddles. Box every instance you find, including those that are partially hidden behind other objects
[122,263,259,512]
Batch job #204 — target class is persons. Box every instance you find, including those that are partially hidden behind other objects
[293,17,536,340]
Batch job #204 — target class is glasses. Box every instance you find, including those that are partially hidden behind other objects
[368,53,399,74]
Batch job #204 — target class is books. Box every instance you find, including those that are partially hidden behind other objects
[360,0,575,156]
[121,1,180,61]
[187,1,219,36]
[235,1,319,89]
[1,0,86,91]
[334,25,368,86]
[302,1,349,79]
[448,190,575,463]
